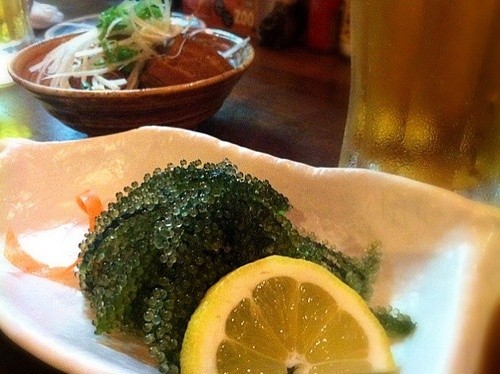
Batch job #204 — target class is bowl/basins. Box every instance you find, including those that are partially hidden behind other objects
[7,26,253,137]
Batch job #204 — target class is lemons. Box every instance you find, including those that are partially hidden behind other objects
[179,255,399,374]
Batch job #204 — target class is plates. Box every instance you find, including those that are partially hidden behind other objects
[45,12,206,39]
[0,125,500,374]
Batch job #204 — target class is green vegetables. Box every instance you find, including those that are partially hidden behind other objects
[93,0,164,73]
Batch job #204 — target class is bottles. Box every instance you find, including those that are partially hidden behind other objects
[0,0,35,87]
[338,0,352,57]
[29,0,64,29]
[339,0,500,206]
[310,0,338,49]
